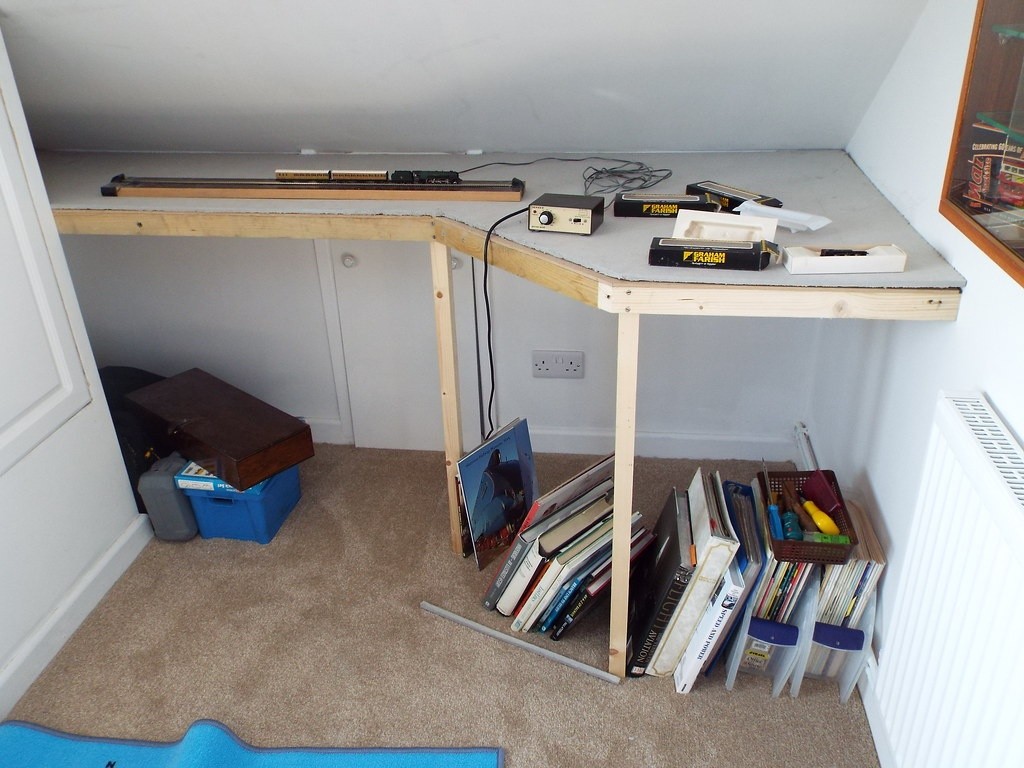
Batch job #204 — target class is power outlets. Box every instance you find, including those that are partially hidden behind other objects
[532,350,584,378]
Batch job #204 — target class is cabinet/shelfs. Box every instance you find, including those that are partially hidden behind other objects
[937,0,1024,289]
[0,28,151,730]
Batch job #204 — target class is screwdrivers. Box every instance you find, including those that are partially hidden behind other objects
[762,457,785,540]
[799,495,840,535]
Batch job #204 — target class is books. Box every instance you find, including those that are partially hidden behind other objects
[480,453,887,694]
[458,418,538,571]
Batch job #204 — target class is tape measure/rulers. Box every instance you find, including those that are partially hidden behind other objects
[101,169,526,201]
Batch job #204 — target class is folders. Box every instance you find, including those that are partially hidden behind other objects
[704,480,767,676]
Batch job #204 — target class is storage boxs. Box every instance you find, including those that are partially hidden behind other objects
[100,365,315,544]
[756,469,859,564]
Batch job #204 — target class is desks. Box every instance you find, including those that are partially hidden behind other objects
[37,146,966,676]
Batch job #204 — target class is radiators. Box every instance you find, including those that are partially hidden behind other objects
[876,384,1024,768]
